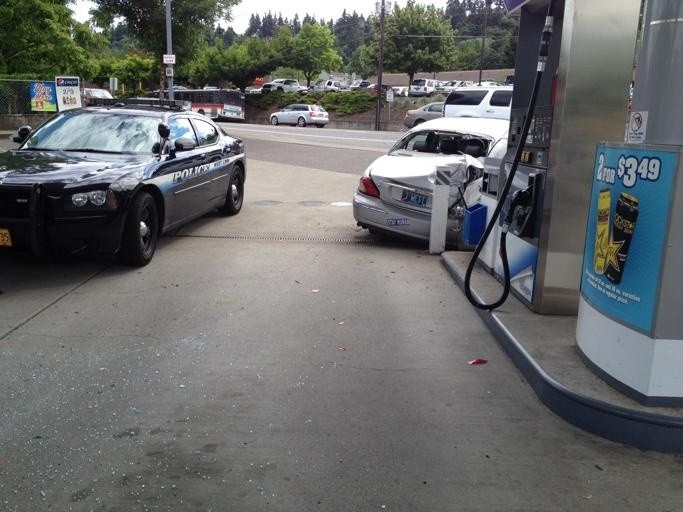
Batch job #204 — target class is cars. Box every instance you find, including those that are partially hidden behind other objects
[0,106,246,265]
[352,114,510,248]
[81,88,114,106]
[403,101,446,129]
[248,74,513,99]
[270,103,329,128]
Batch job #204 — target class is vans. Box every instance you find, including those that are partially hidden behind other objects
[444,85,513,121]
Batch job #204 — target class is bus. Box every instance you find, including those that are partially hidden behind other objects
[152,88,246,122]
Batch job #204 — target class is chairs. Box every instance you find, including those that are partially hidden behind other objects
[413,140,482,157]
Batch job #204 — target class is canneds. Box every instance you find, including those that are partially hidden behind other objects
[603,192,639,285]
[594,188,611,275]
